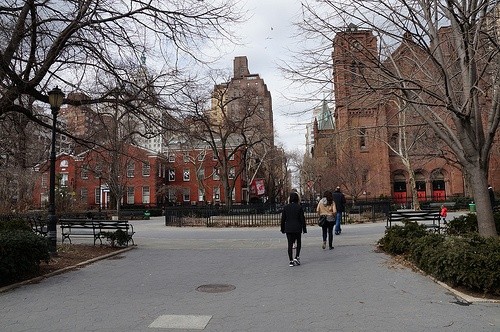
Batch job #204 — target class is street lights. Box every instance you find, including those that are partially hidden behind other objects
[47,85,65,257]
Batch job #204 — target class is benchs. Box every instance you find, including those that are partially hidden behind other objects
[59,218,136,248]
[387,209,441,235]
[429,201,456,212]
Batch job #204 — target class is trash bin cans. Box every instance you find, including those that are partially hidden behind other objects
[469,204,476,212]
[144,210,150,220]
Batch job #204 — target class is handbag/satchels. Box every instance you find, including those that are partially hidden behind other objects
[318,216,326,227]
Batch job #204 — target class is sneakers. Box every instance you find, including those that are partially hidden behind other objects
[292,243,297,248]
[293,257,301,265]
[289,261,294,266]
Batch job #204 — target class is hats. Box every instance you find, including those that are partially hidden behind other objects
[441,205,443,209]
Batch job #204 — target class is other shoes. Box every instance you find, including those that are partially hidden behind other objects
[322,242,326,249]
[339,231,341,233]
[330,247,334,250]
[335,231,339,235]
[443,220,447,224]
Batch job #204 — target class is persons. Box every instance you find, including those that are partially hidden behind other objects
[332,186,345,235]
[317,188,337,250]
[280,193,307,267]
